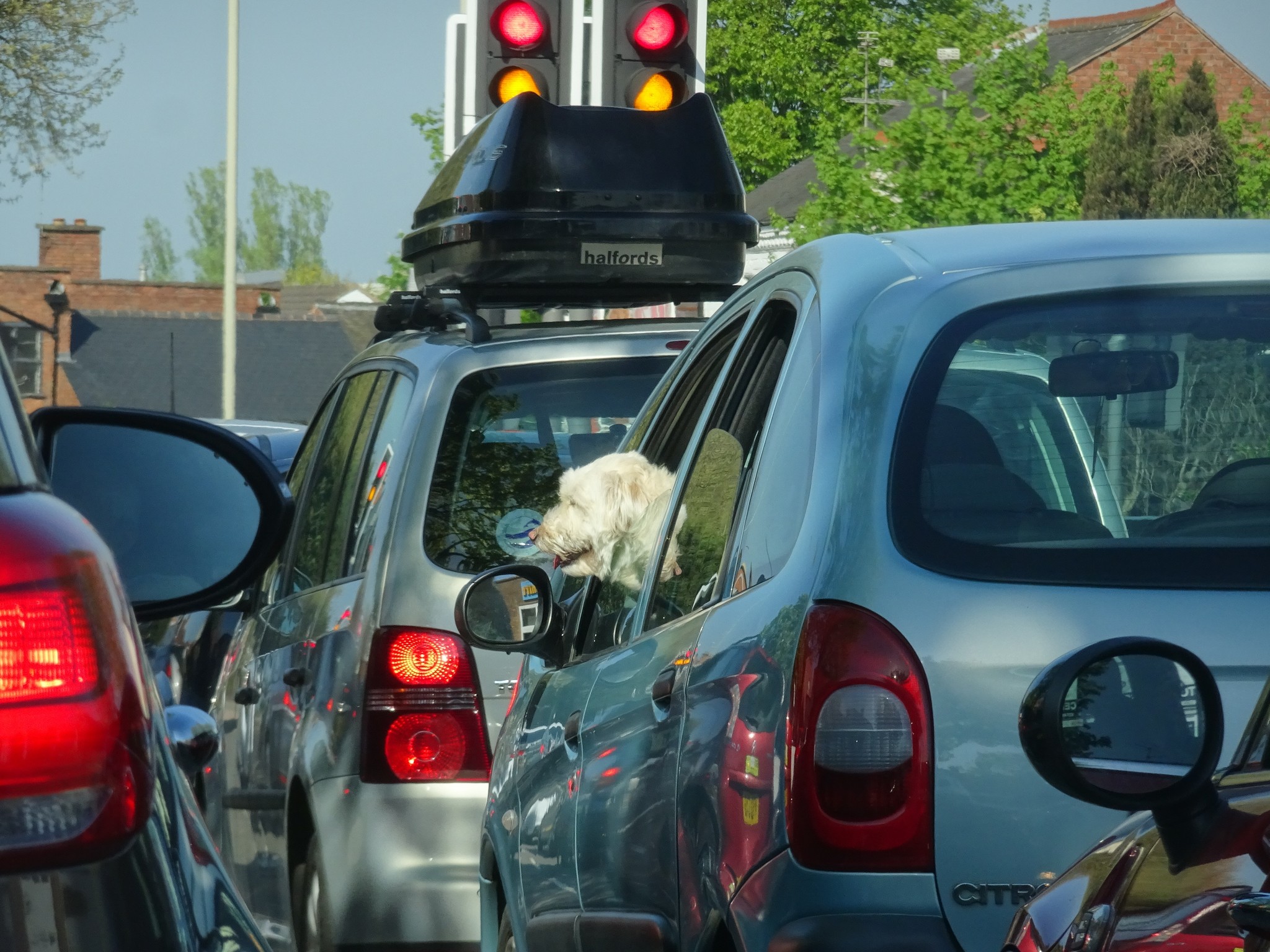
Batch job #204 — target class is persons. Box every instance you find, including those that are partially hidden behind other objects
[169,627,189,705]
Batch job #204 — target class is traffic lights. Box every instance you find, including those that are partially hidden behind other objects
[464,0,582,139]
[589,0,706,114]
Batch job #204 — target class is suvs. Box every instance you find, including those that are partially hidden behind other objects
[199,91,1132,952]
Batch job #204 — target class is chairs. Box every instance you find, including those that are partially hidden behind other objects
[921,404,1111,546]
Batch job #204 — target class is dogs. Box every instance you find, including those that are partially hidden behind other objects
[528,450,681,581]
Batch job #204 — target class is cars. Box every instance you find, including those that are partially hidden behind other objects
[1,313,576,952]
[455,222,1270,951]
[1001,638,1269,952]
